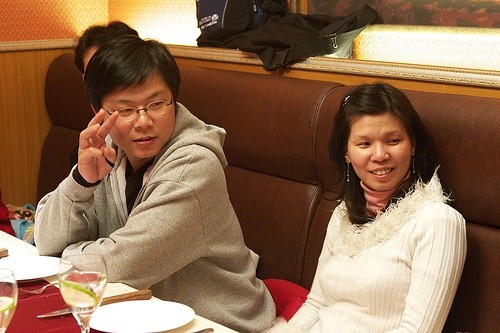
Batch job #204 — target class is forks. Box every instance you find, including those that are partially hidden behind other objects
[20,282,59,293]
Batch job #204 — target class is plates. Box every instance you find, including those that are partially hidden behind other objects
[1,255,73,282]
[87,300,195,333]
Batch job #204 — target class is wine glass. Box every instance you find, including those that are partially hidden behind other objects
[57,252,107,333]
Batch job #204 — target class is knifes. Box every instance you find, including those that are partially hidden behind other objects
[38,290,151,316]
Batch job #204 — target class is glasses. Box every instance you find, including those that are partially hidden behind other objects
[101,98,172,121]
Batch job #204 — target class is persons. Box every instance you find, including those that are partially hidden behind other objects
[69,21,139,171]
[284,82,467,333]
[33,34,276,333]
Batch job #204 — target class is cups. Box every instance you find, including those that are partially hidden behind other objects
[0,270,18,333]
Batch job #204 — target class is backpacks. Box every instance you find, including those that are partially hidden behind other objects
[196,0,288,47]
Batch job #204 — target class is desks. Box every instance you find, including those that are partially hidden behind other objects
[0,200,239,333]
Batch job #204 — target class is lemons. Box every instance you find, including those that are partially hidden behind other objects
[59,280,98,308]
[0,296,15,312]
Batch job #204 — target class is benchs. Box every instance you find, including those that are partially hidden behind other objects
[165,44,500,333]
